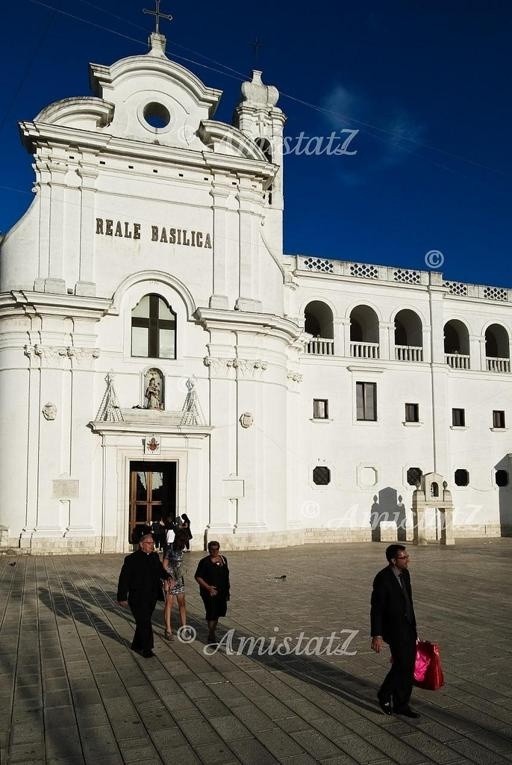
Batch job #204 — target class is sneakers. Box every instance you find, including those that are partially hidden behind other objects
[165,630,174,640]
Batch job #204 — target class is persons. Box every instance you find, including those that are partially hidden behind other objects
[370,545,420,718]
[117,535,177,658]
[146,378,160,408]
[163,534,193,640]
[141,513,192,553]
[194,541,230,651]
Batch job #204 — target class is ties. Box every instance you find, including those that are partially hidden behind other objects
[399,574,411,624]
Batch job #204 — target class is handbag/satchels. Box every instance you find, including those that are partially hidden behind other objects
[413,641,443,690]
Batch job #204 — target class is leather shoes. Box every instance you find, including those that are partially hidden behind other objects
[378,693,391,714]
[394,706,420,718]
